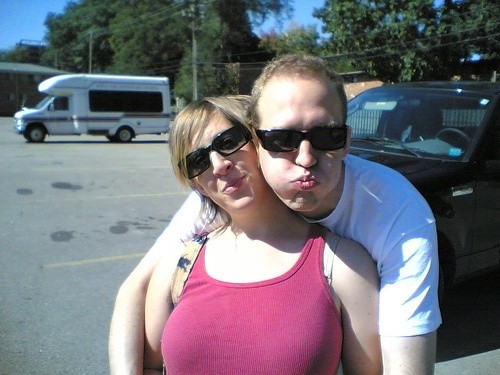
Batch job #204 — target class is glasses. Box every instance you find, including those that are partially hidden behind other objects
[177,123,253,180]
[255,124,348,152]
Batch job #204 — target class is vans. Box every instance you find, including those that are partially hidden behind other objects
[14,75,171,143]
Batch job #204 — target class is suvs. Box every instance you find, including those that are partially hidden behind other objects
[345,82,500,303]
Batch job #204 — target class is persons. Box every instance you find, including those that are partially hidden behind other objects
[109,52,442,375]
[145,93,382,374]
